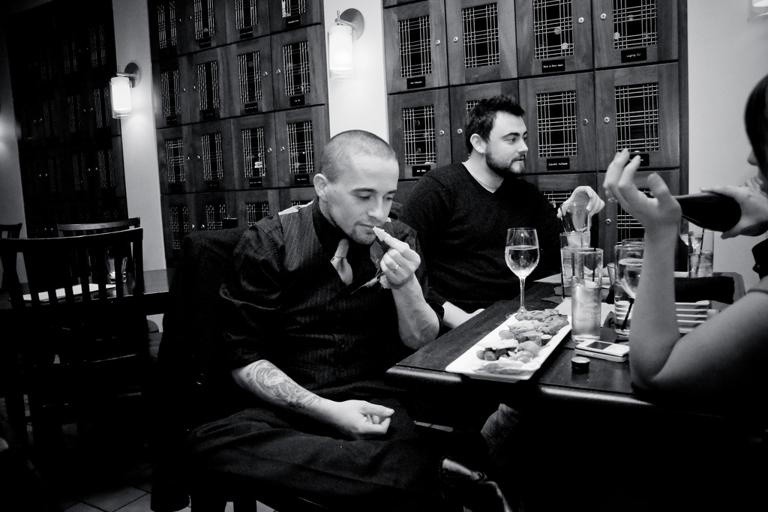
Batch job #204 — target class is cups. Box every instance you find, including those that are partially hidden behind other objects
[606,238,646,297]
[686,229,716,280]
[571,246,604,295]
[568,273,607,344]
[559,231,584,289]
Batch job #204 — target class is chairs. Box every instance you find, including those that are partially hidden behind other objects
[3,219,367,512]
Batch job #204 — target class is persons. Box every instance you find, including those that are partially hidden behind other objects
[207,130,438,512]
[607,74,768,512]
[399,95,605,328]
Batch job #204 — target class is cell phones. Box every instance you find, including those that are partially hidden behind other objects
[575,338,630,363]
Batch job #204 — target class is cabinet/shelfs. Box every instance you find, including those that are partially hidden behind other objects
[146,0,330,248]
[384,2,676,237]
[9,1,126,214]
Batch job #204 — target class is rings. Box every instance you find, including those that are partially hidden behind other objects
[608,196,615,206]
[392,262,397,273]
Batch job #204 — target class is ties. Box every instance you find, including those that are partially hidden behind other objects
[331,239,354,285]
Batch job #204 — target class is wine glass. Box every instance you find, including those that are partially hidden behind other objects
[504,226,541,319]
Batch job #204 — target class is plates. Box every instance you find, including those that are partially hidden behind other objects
[613,298,711,340]
[444,312,572,385]
